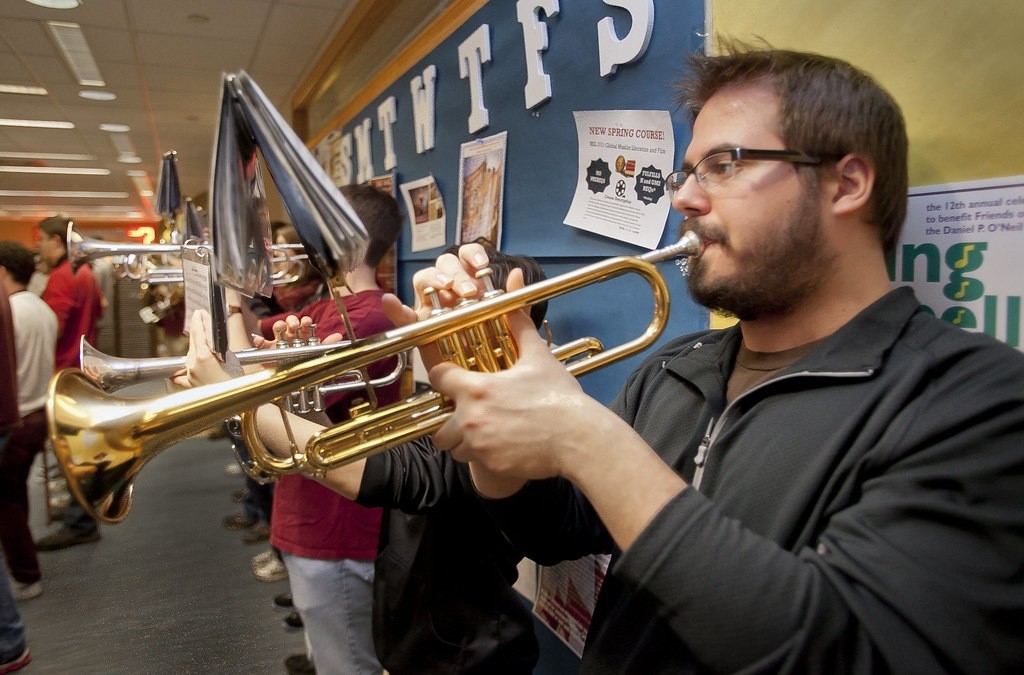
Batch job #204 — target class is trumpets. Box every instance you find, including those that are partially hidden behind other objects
[47,230,705,524]
[66,221,307,286]
[123,249,304,285]
[79,336,407,413]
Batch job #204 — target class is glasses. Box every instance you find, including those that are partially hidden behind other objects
[666,148,883,203]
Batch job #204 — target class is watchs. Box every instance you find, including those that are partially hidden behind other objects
[225,303,244,317]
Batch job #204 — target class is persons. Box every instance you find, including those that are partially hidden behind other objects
[222,183,407,675]
[5,239,59,602]
[33,214,108,553]
[377,30,1024,674]
[171,240,550,675]
[0,276,25,675]
[142,221,329,632]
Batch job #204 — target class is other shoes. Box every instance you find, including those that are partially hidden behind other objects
[285,655,317,675]
[251,550,288,582]
[7,577,43,602]
[223,511,257,530]
[0,646,32,675]
[35,454,101,551]
[208,422,229,440]
[241,488,251,497]
[281,611,303,631]
[272,593,296,611]
[241,522,271,545]
[224,462,243,475]
